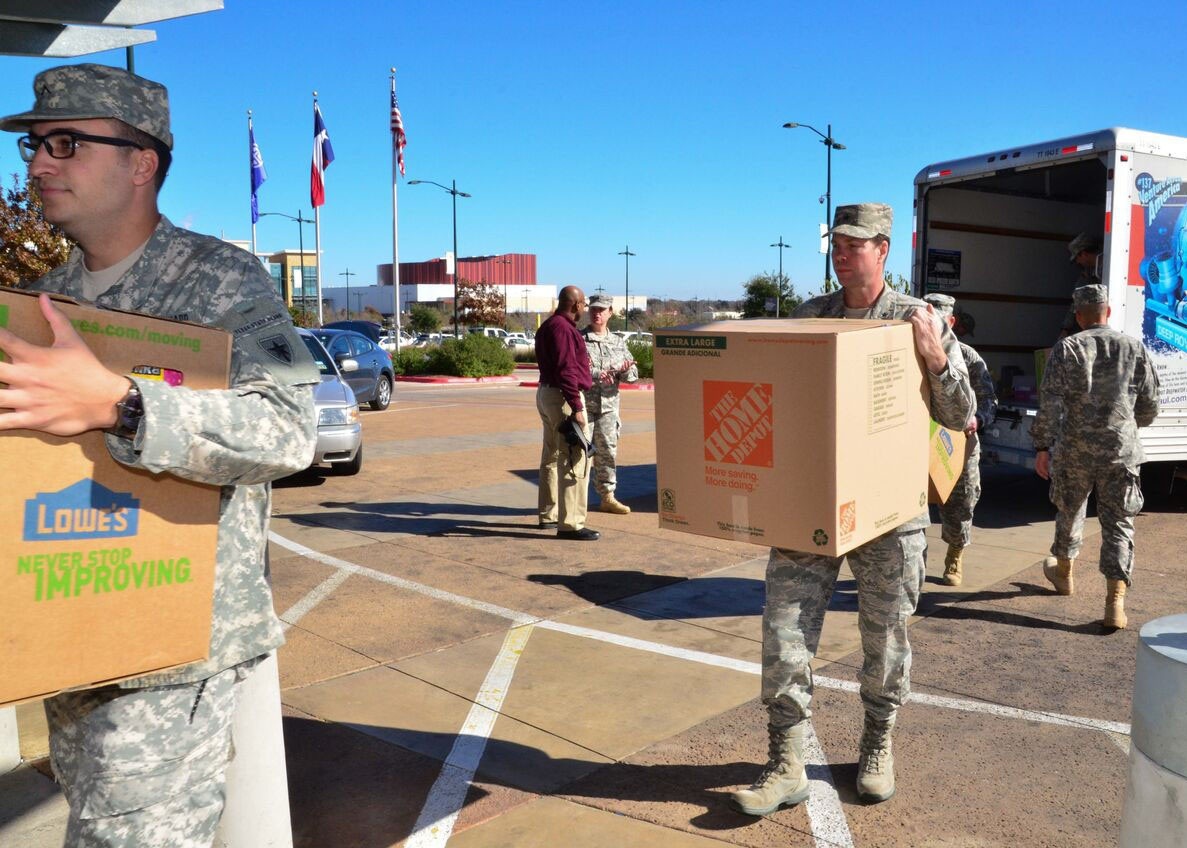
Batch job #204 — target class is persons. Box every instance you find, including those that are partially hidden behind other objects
[953,313,978,344]
[1057,230,1104,341]
[920,290,999,588]
[3,67,322,848]
[578,295,640,514]
[719,203,976,818]
[1032,284,1163,635]
[533,286,600,544]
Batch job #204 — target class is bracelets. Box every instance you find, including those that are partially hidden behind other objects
[1036,446,1050,452]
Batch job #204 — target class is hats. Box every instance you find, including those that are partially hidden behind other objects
[822,202,894,241]
[1070,284,1107,311]
[921,293,955,317]
[1067,232,1094,261]
[588,294,613,309]
[0,64,174,150]
[958,313,976,339]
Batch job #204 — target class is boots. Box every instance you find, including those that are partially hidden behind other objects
[1044,556,1074,594]
[728,724,810,814]
[943,544,964,585]
[599,492,630,513]
[1105,578,1127,629]
[856,713,897,801]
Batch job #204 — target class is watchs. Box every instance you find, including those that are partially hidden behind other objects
[110,383,144,438]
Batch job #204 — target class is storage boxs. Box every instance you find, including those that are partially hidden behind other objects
[925,416,979,505]
[652,310,928,561]
[1,291,234,713]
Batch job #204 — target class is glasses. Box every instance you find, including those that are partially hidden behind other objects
[16,128,144,161]
[578,301,589,306]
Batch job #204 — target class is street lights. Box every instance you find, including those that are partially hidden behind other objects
[339,267,367,320]
[618,245,636,331]
[496,255,511,329]
[784,123,846,293]
[407,179,471,338]
[259,210,315,329]
[595,285,605,293]
[521,288,532,338]
[770,236,790,319]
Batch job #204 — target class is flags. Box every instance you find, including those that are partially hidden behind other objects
[311,103,336,210]
[389,79,408,180]
[248,124,268,225]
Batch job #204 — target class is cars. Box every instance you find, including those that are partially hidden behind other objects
[612,331,653,346]
[294,320,394,475]
[377,327,535,357]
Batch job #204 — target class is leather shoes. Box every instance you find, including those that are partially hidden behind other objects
[557,527,600,539]
[540,521,559,528]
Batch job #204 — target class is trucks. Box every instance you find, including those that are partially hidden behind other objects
[910,127,1187,509]
